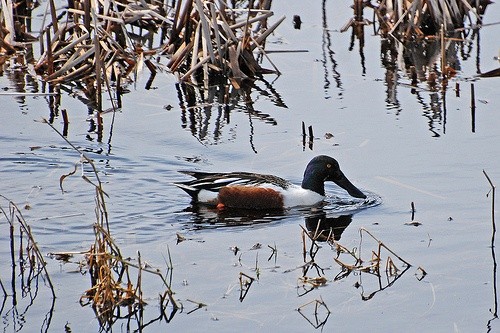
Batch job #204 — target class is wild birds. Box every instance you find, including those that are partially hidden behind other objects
[172,155,368,213]
